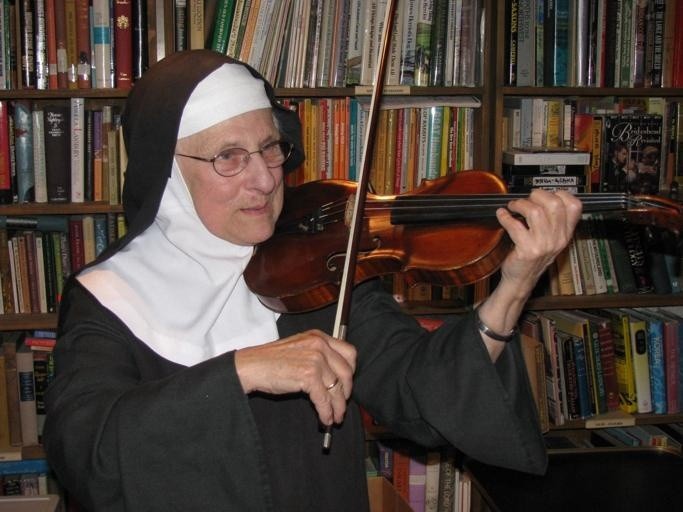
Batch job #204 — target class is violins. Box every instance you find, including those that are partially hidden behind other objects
[242,169,679,314]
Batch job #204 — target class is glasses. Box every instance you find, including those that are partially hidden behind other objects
[176,137,294,177]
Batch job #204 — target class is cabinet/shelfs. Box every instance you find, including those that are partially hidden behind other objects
[165,0,493,512]
[494,1,683,511]
[1,1,164,512]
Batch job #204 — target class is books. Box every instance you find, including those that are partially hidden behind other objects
[276,96,483,196]
[0,94,130,208]
[519,306,682,436]
[0,473,59,497]
[0,0,150,91]
[1,327,57,461]
[505,0,683,89]
[174,1,484,90]
[501,94,682,197]
[368,435,472,512]
[546,216,682,295]
[1,210,127,316]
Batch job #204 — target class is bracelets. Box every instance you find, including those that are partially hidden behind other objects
[476,309,517,342]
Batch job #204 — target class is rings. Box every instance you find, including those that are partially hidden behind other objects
[325,377,339,391]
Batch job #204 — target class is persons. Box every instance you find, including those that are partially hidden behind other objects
[40,48,585,512]
